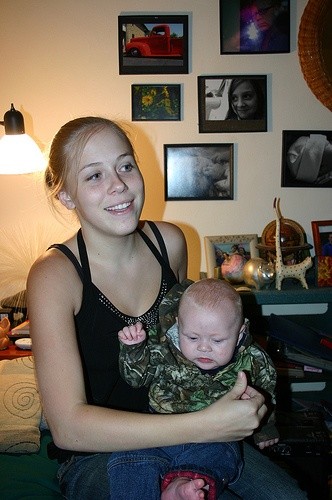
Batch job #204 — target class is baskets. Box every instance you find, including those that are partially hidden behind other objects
[298,0,332,112]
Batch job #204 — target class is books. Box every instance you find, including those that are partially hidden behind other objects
[289,380,325,393]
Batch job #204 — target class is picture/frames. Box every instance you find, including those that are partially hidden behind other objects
[205,234,260,280]
[163,143,234,202]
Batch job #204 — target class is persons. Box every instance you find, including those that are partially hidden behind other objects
[215,243,249,266]
[105,278,281,500]
[225,78,266,119]
[241,2,289,52]
[285,132,332,187]
[27,117,308,499]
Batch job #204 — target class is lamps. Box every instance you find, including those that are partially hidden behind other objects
[0,102,47,176]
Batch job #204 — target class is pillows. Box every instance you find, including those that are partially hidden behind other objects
[0,356,42,454]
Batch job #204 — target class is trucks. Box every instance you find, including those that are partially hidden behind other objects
[126,24,185,58]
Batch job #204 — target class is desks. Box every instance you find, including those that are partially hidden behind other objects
[252,289,332,442]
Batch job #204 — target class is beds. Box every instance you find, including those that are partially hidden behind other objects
[0,289,61,500]
